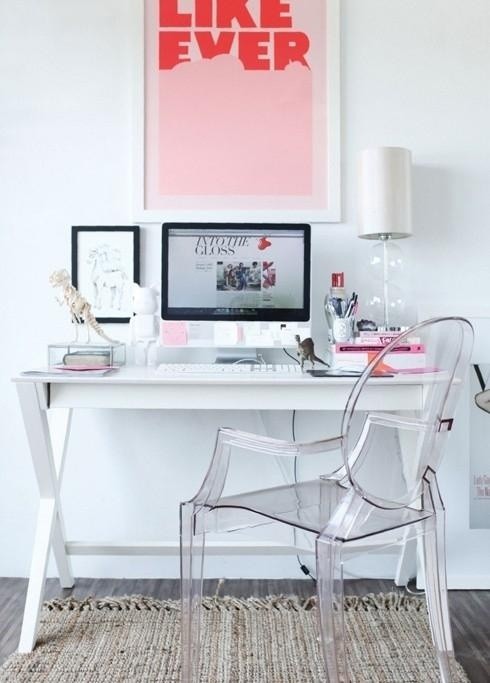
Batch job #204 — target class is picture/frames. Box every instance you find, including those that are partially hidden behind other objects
[417,316,490,590]
[134,0,342,224]
[73,227,139,324]
[160,224,312,323]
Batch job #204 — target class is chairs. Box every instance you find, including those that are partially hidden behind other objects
[181,340,474,682]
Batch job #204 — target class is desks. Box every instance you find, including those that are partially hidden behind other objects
[10,364,463,652]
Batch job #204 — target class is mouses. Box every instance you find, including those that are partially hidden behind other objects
[338,363,370,374]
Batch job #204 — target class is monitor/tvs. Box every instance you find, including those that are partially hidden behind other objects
[157,220,312,362]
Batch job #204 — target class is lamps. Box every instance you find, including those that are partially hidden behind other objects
[360,146,413,332]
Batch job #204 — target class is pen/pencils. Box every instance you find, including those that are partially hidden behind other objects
[326,292,359,319]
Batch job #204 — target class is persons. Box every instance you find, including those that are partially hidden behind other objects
[216,261,260,290]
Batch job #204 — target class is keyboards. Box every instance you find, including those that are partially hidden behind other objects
[155,364,303,379]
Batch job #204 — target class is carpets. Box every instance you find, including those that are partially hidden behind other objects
[2,594,471,682]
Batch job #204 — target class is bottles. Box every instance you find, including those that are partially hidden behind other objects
[325,272,351,330]
